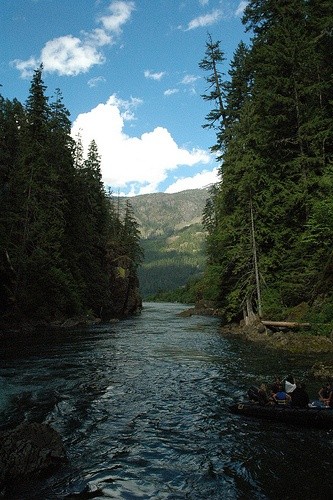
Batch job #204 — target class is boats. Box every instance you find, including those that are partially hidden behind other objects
[226,394,333,428]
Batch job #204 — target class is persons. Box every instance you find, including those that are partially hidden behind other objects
[284,374,297,400]
[272,389,291,404]
[248,376,281,405]
[288,383,310,408]
[318,380,333,401]
